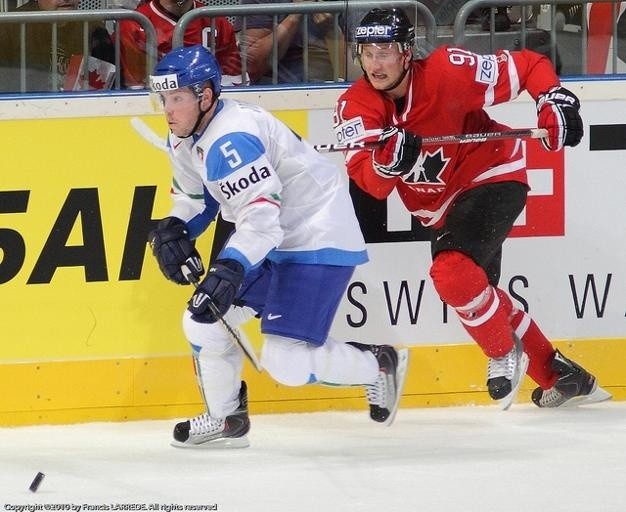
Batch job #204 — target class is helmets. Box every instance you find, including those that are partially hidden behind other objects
[149,45,221,102]
[352,8,414,65]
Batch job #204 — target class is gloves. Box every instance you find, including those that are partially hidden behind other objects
[148,217,205,285]
[187,257,244,324]
[534,85,585,152]
[371,126,422,179]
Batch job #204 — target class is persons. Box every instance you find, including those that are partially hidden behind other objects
[147,44,398,444]
[334,8,597,409]
[1,0,626,93]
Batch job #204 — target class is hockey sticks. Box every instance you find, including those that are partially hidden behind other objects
[130,117,548,150]
[181,265,261,372]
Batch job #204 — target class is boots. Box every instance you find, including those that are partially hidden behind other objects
[174,379,249,445]
[488,331,523,399]
[346,341,397,421]
[531,348,597,407]
